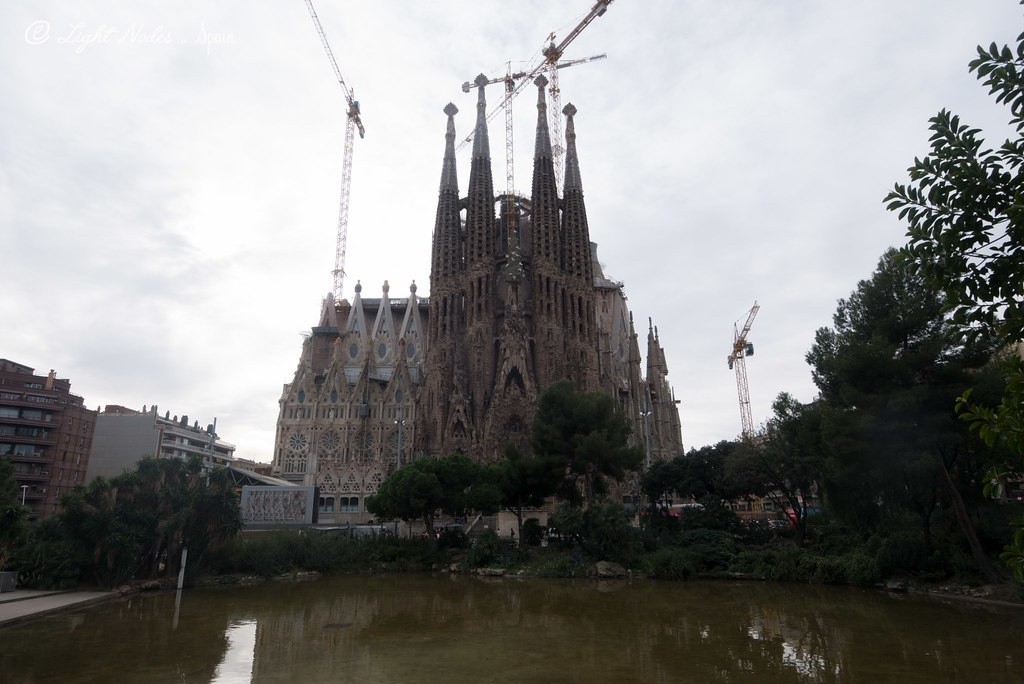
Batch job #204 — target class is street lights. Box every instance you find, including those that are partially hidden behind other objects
[19,484,29,506]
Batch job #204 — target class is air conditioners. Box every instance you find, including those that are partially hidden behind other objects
[41,472,48,477]
[33,453,41,458]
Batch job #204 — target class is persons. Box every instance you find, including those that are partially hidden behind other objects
[411,522,514,540]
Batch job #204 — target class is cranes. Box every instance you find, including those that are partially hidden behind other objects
[726,300,761,441]
[454,0,612,196]
[304,0,367,312]
